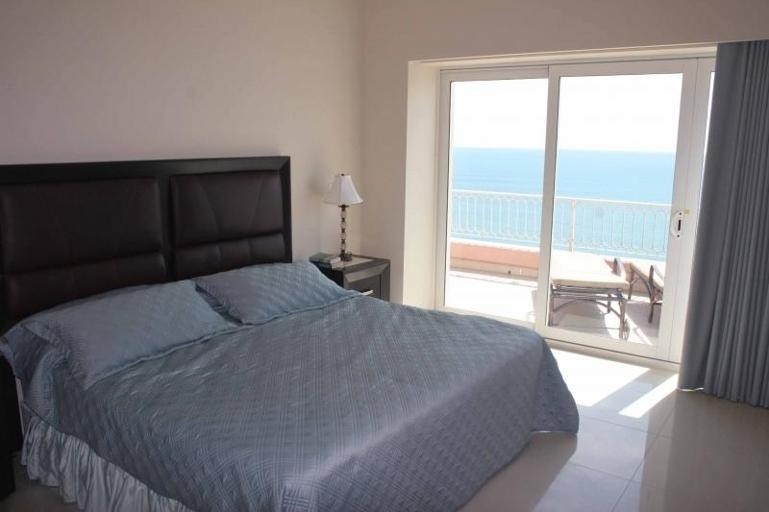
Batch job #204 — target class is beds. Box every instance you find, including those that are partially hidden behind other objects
[0,156,545,510]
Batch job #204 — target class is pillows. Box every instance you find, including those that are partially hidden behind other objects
[195,260,370,330]
[20,276,236,393]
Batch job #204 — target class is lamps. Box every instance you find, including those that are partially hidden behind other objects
[321,171,363,261]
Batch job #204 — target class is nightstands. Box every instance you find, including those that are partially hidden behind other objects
[310,251,390,302]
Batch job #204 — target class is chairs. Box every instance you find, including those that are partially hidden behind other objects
[547,249,669,340]
